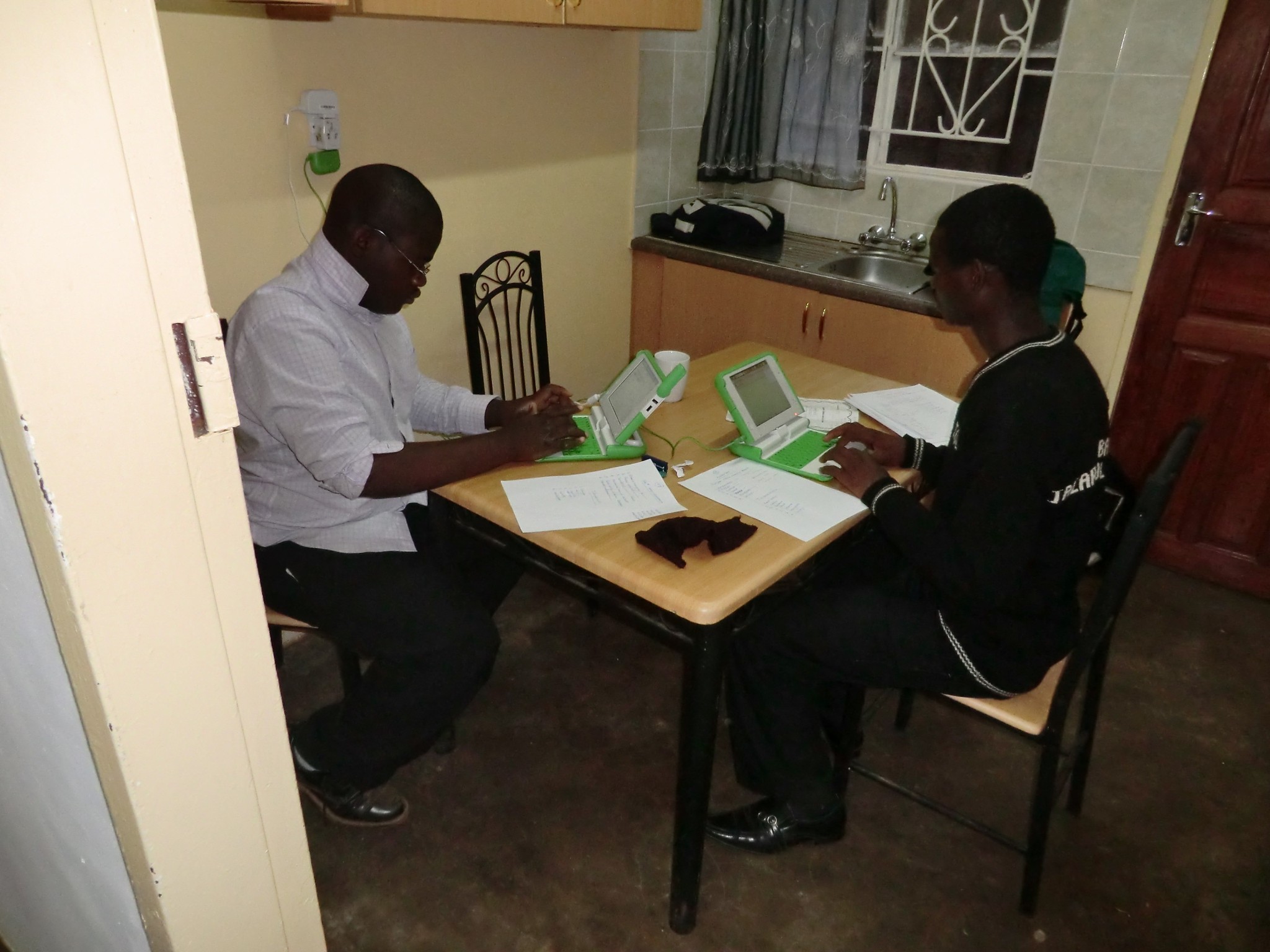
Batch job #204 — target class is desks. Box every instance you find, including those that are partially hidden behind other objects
[431,340,963,934]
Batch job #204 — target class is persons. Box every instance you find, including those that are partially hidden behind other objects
[227,163,587,829]
[709,183,1113,856]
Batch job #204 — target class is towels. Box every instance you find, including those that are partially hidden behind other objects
[650,197,785,245]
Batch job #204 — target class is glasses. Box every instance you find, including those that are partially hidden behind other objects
[370,229,431,280]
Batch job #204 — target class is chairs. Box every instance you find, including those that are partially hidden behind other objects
[458,248,552,397]
[846,411,1205,911]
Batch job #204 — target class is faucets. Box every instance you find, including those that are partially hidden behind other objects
[858,176,927,253]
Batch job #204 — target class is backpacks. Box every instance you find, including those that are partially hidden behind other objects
[649,196,786,262]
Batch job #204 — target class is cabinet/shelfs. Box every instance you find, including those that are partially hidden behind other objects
[630,249,966,392]
[356,0,702,29]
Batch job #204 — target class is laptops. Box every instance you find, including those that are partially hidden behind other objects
[534,350,686,462]
[715,352,867,482]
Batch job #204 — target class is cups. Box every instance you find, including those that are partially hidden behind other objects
[654,350,691,402]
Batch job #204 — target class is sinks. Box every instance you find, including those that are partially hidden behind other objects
[818,256,934,295]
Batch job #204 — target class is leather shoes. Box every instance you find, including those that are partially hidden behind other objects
[707,794,846,849]
[290,726,407,827]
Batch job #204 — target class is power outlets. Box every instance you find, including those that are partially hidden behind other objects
[309,117,339,146]
